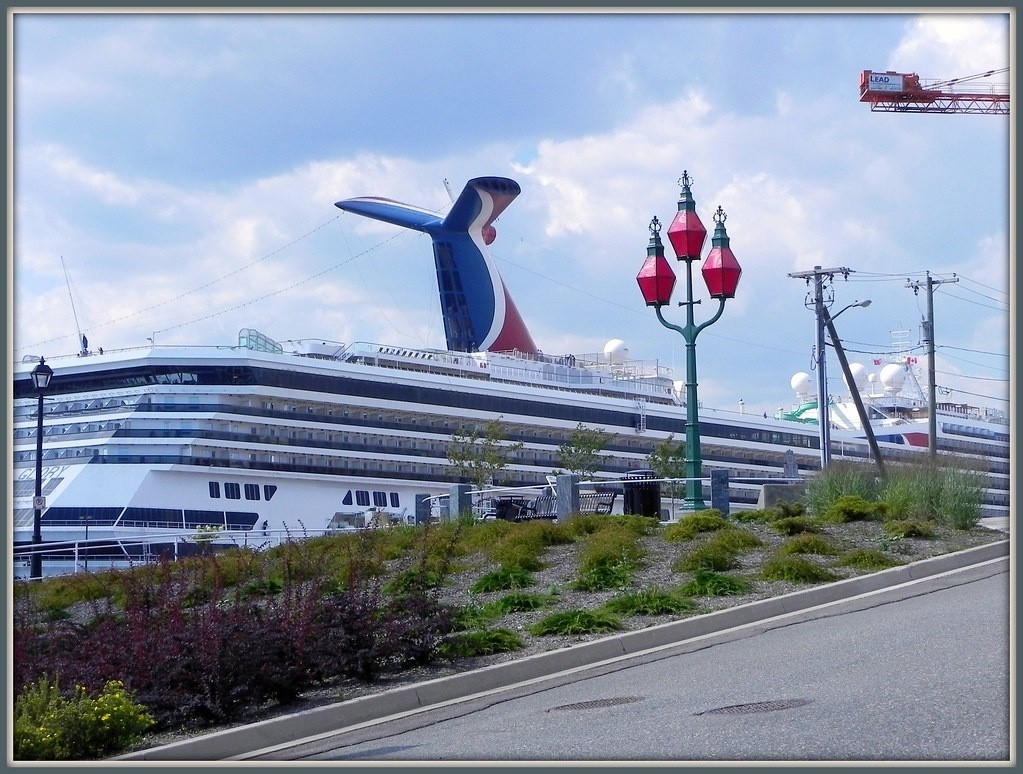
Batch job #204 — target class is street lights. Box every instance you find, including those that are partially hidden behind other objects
[30,356,55,582]
[634,168,743,516]
[809,298,873,475]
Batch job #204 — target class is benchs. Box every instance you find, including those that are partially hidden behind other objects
[483,491,618,521]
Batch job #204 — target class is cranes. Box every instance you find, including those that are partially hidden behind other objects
[858,65,1010,115]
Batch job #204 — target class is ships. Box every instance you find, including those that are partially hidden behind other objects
[10,174,1009,579]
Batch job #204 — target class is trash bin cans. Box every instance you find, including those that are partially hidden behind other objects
[619,469,661,518]
